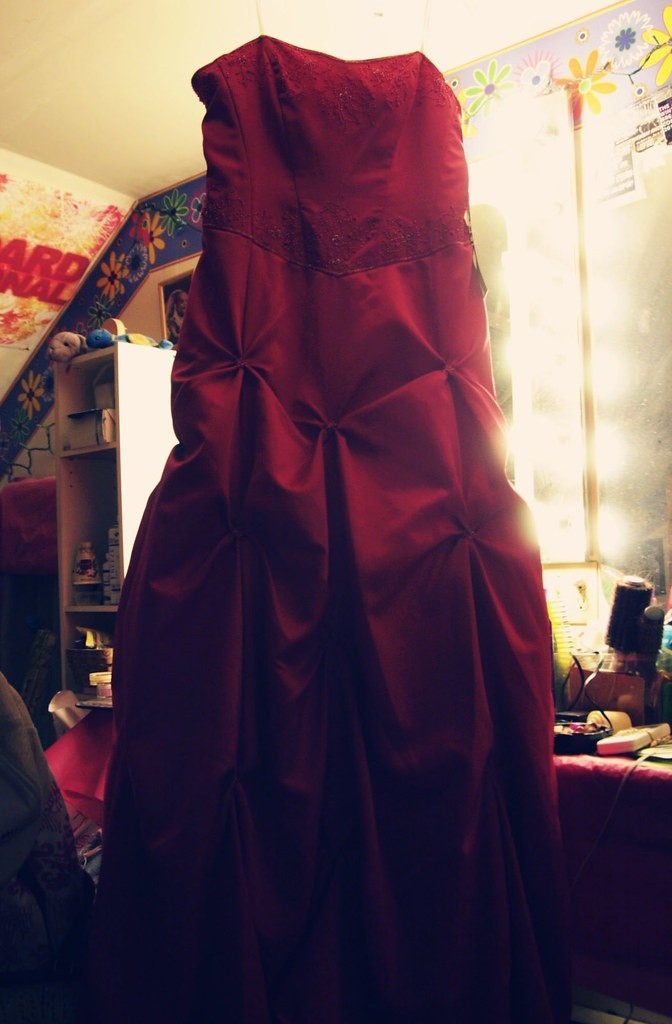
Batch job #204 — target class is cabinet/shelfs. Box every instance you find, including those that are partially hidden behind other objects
[50,338,181,695]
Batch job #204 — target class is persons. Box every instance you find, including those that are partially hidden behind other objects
[1,672,90,1024]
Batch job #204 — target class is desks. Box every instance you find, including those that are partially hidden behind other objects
[42,698,672,1007]
